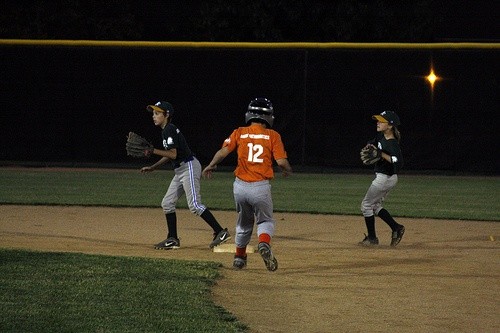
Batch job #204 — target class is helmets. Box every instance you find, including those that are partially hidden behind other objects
[245,98,275,128]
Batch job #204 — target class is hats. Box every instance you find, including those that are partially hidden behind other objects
[372,111,400,126]
[147,100,174,115]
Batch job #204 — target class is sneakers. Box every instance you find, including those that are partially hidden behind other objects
[390,225,405,248]
[209,228,230,248]
[153,238,181,251]
[358,234,379,246]
[257,241,278,272]
[233,254,248,269]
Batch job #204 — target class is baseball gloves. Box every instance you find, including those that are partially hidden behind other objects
[360,144,382,164]
[126,130,154,159]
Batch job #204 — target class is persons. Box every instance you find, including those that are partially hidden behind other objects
[201,97,292,272]
[139,101,233,251]
[355,110,406,248]
